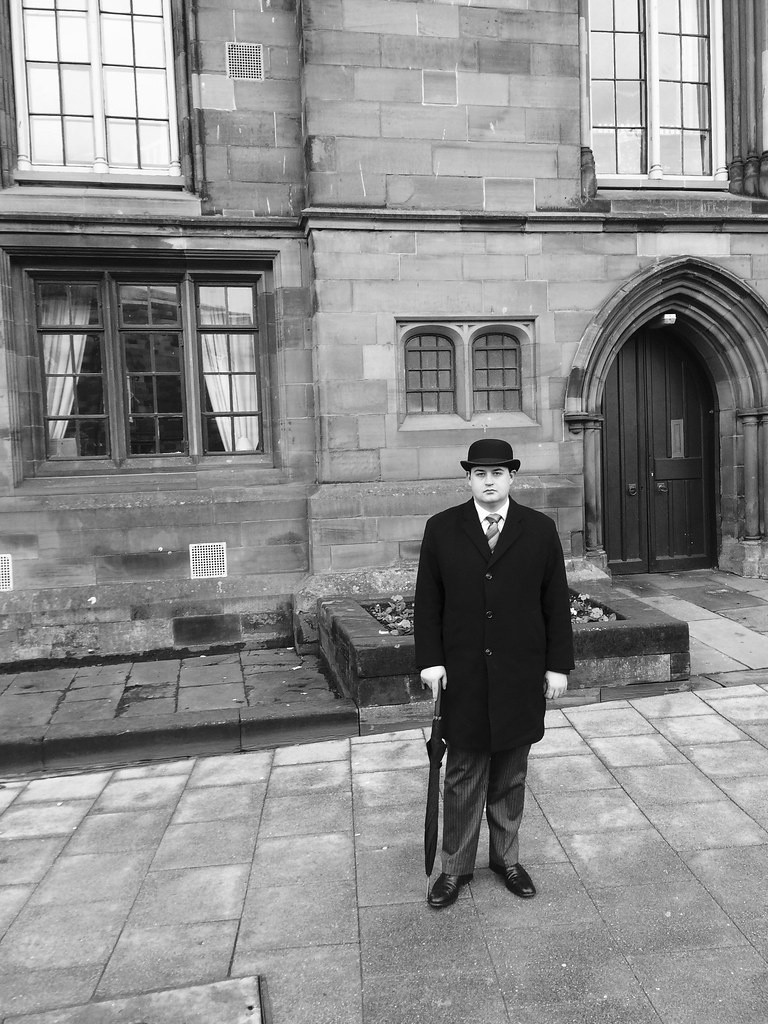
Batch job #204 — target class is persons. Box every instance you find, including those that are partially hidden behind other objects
[415,439,575,908]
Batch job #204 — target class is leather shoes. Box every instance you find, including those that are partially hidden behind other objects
[489,863,536,898]
[430,872,473,907]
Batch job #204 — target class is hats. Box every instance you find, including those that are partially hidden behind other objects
[460,439,521,475]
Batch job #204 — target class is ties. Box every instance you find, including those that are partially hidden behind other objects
[486,514,501,554]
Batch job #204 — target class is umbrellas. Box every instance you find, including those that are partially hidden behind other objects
[421,678,447,902]
